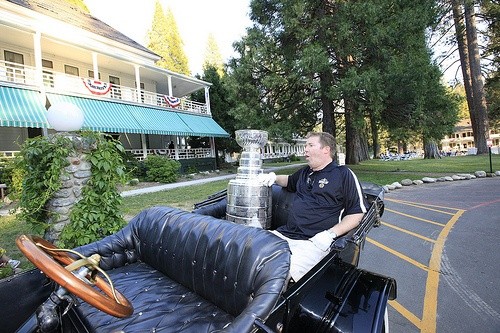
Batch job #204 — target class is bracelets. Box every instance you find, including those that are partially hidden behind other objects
[269,172,277,180]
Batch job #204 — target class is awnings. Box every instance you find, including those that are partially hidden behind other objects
[0,81,230,137]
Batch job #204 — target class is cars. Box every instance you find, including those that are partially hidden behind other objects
[0,182,399,332]
[380,150,424,161]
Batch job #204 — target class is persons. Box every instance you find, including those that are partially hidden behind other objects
[379,150,459,158]
[259,132,368,282]
[169,141,175,156]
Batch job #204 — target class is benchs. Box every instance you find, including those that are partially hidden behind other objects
[65,206,292,333]
[192,183,385,249]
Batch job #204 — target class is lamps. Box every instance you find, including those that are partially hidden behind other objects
[46,102,83,132]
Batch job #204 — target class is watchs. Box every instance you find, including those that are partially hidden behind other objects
[327,229,337,239]
